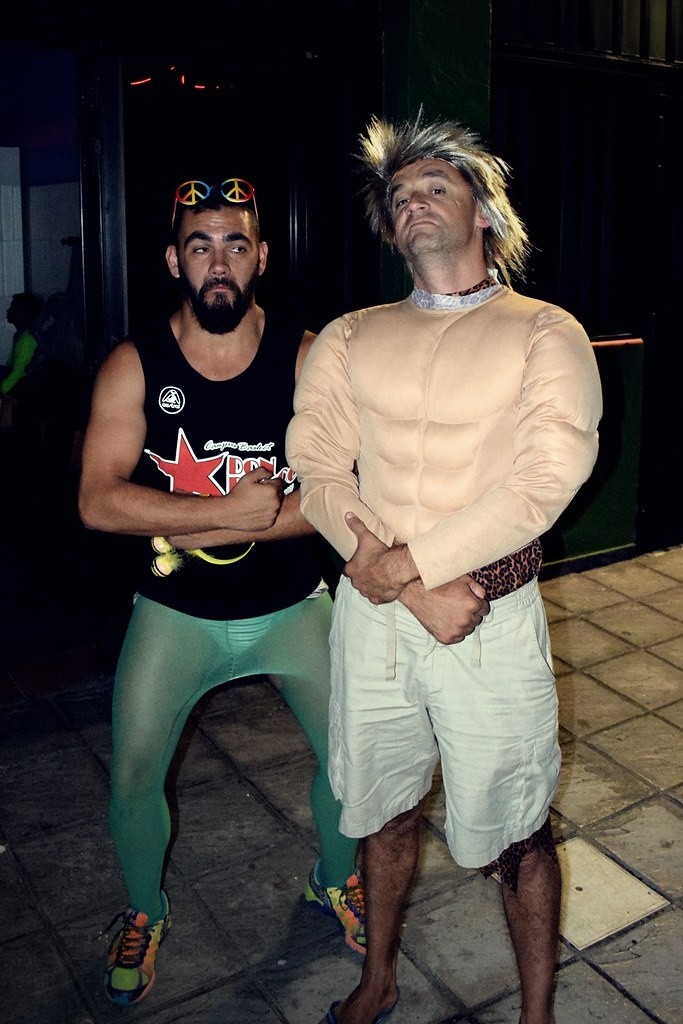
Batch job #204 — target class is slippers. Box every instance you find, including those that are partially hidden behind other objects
[319,986,400,1024]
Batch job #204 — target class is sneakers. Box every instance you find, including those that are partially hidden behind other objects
[305,860,372,955]
[105,889,171,1003]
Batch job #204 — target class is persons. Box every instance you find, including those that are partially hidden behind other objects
[274,108,606,1024]
[74,166,373,1006]
[0,291,56,459]
